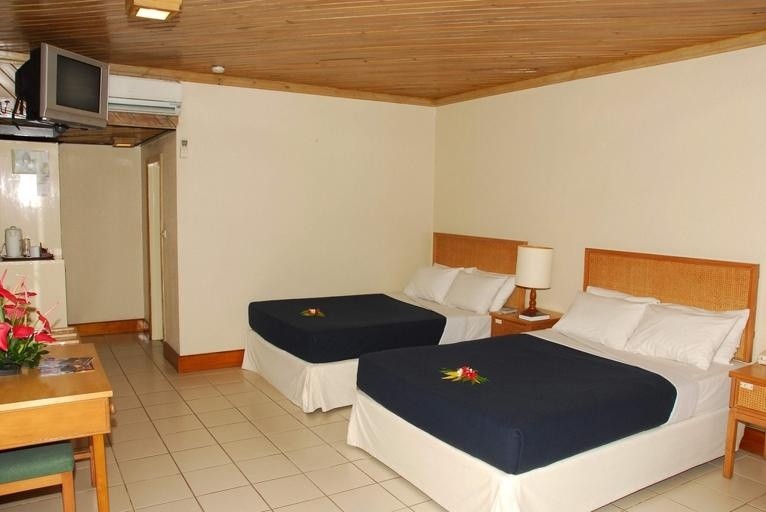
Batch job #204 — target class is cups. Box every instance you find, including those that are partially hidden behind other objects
[30,246,40,257]
[20,237,31,258]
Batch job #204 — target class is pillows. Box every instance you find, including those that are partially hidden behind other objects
[585,285,662,305]
[432,261,478,274]
[552,291,645,351]
[442,270,511,316]
[665,304,751,363]
[472,269,516,312]
[404,264,465,304]
[623,303,741,373]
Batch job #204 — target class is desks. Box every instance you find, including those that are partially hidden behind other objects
[0,341,115,512]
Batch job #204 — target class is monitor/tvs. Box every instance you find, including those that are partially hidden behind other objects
[15,42,107,129]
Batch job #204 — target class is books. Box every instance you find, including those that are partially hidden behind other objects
[519,314,550,321]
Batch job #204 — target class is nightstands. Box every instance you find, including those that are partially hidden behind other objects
[723,362,765,479]
[489,309,563,336]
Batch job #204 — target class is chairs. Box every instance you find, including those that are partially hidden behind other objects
[1,445,77,512]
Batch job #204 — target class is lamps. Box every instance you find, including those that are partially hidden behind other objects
[514,245,554,314]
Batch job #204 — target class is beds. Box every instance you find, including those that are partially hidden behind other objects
[242,233,529,417]
[344,246,759,512]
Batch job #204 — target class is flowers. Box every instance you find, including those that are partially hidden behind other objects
[0,268,59,367]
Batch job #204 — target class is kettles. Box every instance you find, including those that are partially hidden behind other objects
[4,226,23,258]
